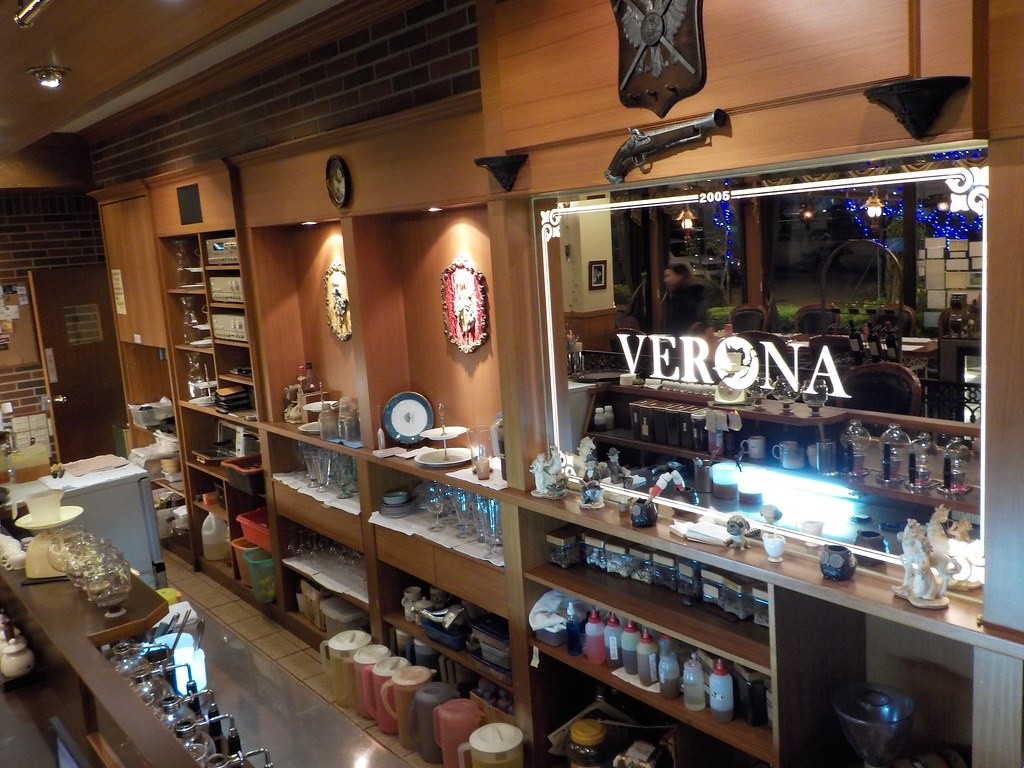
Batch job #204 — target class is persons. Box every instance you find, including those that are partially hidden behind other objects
[661,262,723,330]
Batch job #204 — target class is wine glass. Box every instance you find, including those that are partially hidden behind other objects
[298,441,503,560]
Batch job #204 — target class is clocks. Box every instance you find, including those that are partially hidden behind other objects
[325,154,352,208]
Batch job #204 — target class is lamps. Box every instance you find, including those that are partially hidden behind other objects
[25,67,72,88]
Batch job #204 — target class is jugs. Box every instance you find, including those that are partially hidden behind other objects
[319,630,528,768]
[10,488,64,524]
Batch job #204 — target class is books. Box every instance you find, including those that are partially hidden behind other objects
[214,386,252,413]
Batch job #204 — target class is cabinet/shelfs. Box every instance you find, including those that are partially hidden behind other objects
[86,90,980,768]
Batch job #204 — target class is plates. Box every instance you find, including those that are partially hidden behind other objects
[383,391,472,468]
[188,380,217,406]
[180,267,212,348]
[297,399,338,434]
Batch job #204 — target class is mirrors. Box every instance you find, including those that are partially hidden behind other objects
[531,139,988,578]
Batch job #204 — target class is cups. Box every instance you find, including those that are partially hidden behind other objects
[0,533,26,570]
[772,441,797,462]
[401,583,453,627]
[695,460,715,493]
[741,435,767,459]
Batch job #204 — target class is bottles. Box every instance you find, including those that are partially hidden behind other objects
[171,240,202,345]
[530,372,975,567]
[108,642,232,768]
[319,397,360,442]
[296,361,315,392]
[185,352,206,398]
[489,412,507,480]
[565,719,607,768]
[566,603,733,726]
[0,525,130,677]
[846,319,900,364]
[202,512,227,561]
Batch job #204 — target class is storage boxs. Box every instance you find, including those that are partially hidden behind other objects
[220,454,265,496]
[128,401,175,428]
[421,618,464,650]
[236,508,273,552]
[469,688,516,727]
